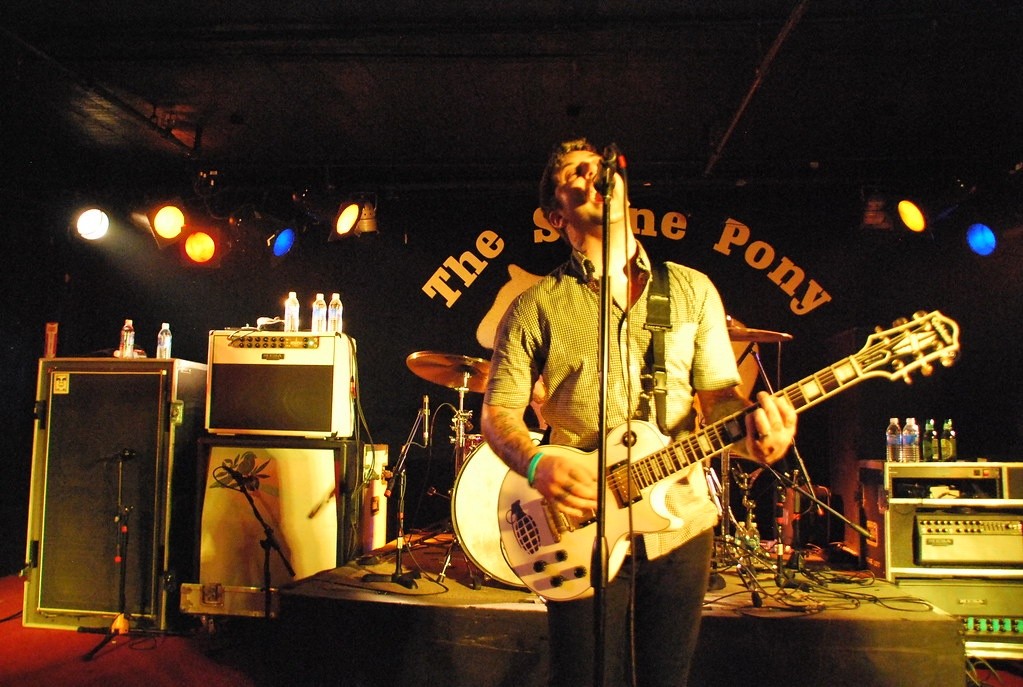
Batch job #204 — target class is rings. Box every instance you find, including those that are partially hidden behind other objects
[763,445,775,456]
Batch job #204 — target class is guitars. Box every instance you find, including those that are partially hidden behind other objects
[495,305,965,605]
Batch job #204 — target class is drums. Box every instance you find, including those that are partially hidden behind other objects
[449,429,630,596]
[454,431,485,476]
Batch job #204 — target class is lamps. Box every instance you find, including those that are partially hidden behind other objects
[965,204,1023,256]
[246,213,295,258]
[310,191,362,238]
[894,173,964,235]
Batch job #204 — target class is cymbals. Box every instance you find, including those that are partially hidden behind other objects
[405,350,493,394]
[729,326,793,344]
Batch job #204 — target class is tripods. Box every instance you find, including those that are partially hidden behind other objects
[78,459,192,662]
[363,409,424,590]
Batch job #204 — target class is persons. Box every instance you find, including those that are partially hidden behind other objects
[481,137,797,687]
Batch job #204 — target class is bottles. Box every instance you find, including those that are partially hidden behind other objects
[157,323,172,359]
[327,292,343,335]
[283,291,300,333]
[886,418,902,462]
[902,418,919,462]
[923,419,939,462]
[120,320,135,358]
[941,418,956,462]
[747,523,761,565]
[44,321,59,359]
[735,521,749,568]
[759,539,791,554]
[312,293,326,335]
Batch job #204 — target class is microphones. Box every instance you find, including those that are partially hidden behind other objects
[423,394,430,446]
[736,341,756,367]
[593,146,615,195]
[117,447,138,459]
[221,465,251,483]
[448,404,474,432]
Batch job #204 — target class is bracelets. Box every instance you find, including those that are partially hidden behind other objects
[529,453,544,484]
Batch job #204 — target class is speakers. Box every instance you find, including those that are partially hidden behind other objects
[194,436,389,588]
[204,329,357,440]
[26,357,209,633]
